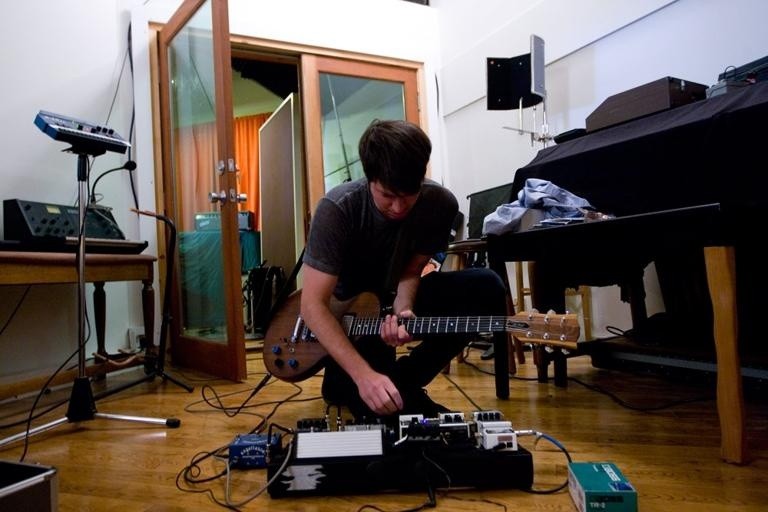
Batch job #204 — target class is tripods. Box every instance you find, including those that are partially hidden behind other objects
[0,150,180,448]
[92,213,194,400]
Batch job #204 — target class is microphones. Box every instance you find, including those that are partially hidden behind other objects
[91,161,136,204]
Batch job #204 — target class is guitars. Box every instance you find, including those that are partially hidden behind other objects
[262,288,580,381]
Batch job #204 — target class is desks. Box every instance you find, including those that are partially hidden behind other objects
[449,238,592,363]
[0,246,157,401]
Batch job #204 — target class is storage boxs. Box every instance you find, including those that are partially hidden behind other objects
[1,458,58,512]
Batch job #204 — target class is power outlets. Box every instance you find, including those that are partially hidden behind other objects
[128,325,145,349]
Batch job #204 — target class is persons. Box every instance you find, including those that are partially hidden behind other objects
[300,119,509,419]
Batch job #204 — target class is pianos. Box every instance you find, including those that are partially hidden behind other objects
[485,80,768,464]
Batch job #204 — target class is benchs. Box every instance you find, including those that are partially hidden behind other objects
[485,203,768,465]
